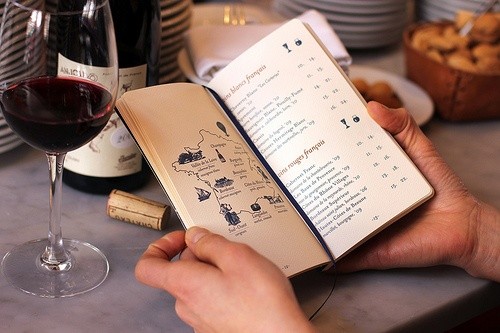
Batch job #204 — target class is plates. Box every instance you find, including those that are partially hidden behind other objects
[272,0,500,49]
[177,49,432,126]
[159,0,193,85]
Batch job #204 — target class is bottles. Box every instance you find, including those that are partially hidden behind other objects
[47,0,162,195]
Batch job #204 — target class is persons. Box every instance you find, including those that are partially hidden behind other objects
[133,99,500,333]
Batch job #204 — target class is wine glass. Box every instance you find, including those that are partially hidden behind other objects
[0,0,119,297]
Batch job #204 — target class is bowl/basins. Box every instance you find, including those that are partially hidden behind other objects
[403,19,500,122]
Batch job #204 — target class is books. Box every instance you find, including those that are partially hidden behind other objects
[113,18,438,282]
